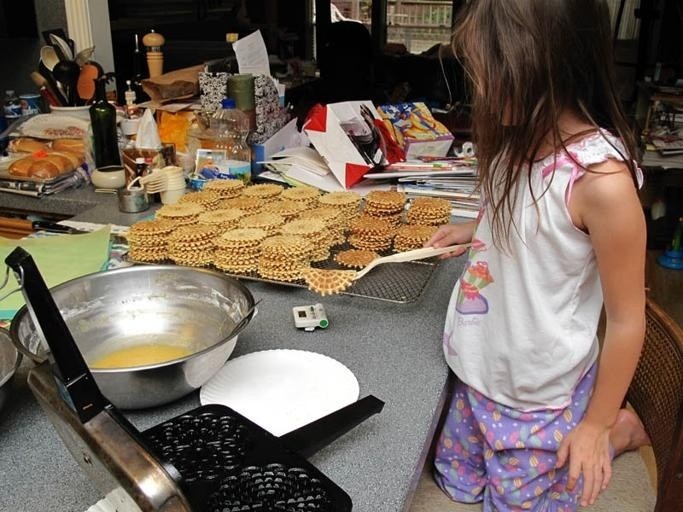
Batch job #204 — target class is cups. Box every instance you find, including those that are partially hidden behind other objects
[50,104,92,120]
[19,94,42,115]
[140,166,208,205]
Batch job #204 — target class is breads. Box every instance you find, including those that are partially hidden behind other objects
[8,138,85,179]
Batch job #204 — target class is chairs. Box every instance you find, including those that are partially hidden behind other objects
[404,286,683,509]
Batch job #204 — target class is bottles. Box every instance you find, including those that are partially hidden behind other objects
[89,78,146,189]
[210,99,251,190]
[3,89,21,127]
[672,213,682,252]
[654,62,663,83]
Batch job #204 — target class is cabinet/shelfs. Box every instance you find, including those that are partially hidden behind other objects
[631,75,683,207]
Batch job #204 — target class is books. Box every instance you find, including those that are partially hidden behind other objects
[647,86,683,158]
[364,153,481,219]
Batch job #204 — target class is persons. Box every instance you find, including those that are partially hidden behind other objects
[417,0,653,511]
[359,103,405,163]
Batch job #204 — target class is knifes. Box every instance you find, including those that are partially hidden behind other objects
[32,220,128,244]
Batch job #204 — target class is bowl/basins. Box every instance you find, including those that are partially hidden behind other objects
[0,264,255,408]
[118,185,151,213]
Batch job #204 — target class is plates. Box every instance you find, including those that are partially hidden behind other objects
[201,349,359,437]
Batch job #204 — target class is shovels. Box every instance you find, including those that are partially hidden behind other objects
[42,29,66,46]
[352,243,471,280]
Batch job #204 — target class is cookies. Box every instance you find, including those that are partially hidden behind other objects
[128,178,451,296]
